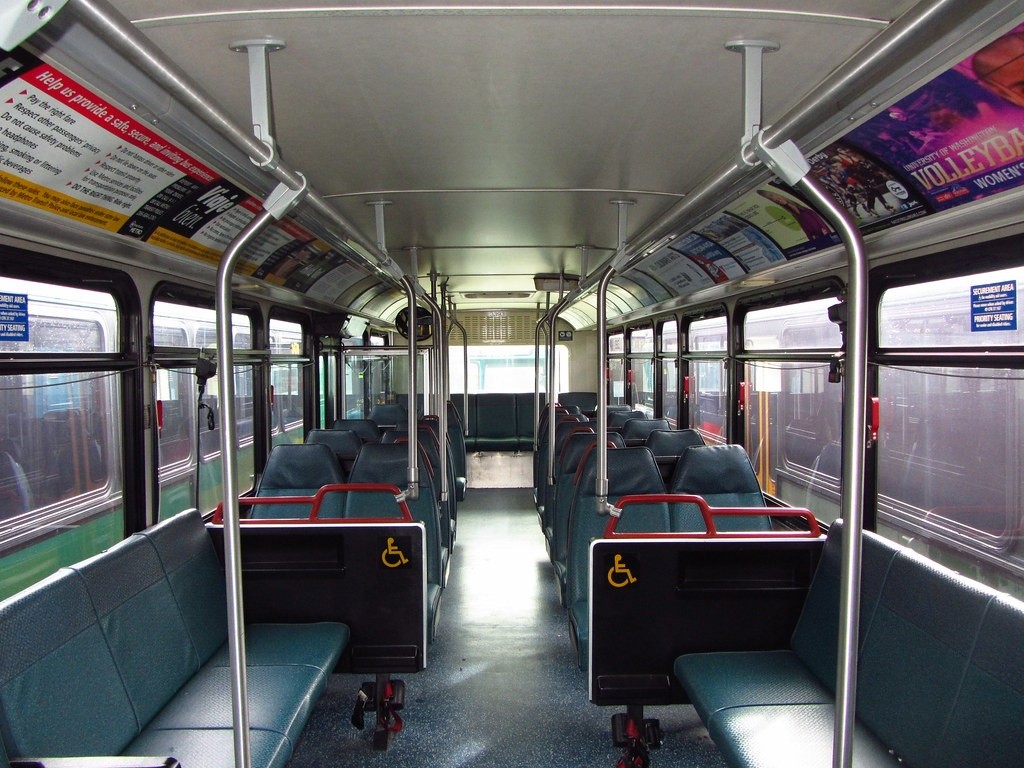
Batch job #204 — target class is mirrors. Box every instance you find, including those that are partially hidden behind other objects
[395,304,434,342]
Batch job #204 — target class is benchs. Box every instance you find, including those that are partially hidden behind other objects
[392,393,598,453]
[674,518,1024,768]
[0,507,348,768]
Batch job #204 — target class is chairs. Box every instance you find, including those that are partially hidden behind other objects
[394,416,457,555]
[419,400,465,501]
[251,443,346,522]
[346,434,441,644]
[642,429,706,492]
[595,404,631,418]
[623,418,672,447]
[331,419,380,444]
[305,429,362,477]
[0,394,104,526]
[607,410,647,429]
[544,416,597,558]
[538,409,587,529]
[367,402,409,427]
[672,443,774,533]
[566,441,672,671]
[381,427,450,589]
[552,426,627,609]
[532,402,582,510]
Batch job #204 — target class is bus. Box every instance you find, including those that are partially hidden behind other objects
[613,281,1024,605]
[0,276,363,600]
